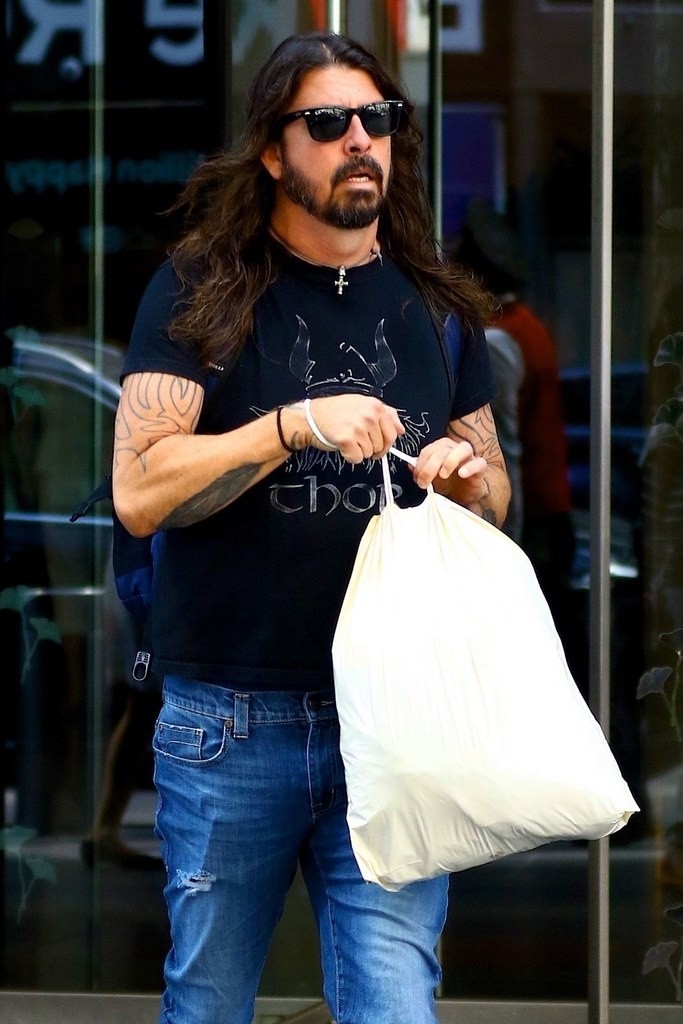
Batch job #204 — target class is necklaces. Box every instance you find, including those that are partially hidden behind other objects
[268,227,376,295]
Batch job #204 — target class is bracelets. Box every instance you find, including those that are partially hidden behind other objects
[276,406,303,453]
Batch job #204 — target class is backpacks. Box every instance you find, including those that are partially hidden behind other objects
[112,272,465,682]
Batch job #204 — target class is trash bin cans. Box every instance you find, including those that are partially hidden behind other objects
[19,585,98,832]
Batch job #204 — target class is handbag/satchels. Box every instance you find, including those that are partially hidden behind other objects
[332,446,645,897]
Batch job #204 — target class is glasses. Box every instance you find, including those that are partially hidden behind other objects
[268,98,407,144]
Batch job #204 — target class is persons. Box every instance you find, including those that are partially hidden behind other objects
[112,29,512,1024]
[453,208,573,664]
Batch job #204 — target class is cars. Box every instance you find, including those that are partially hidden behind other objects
[4,334,640,593]
